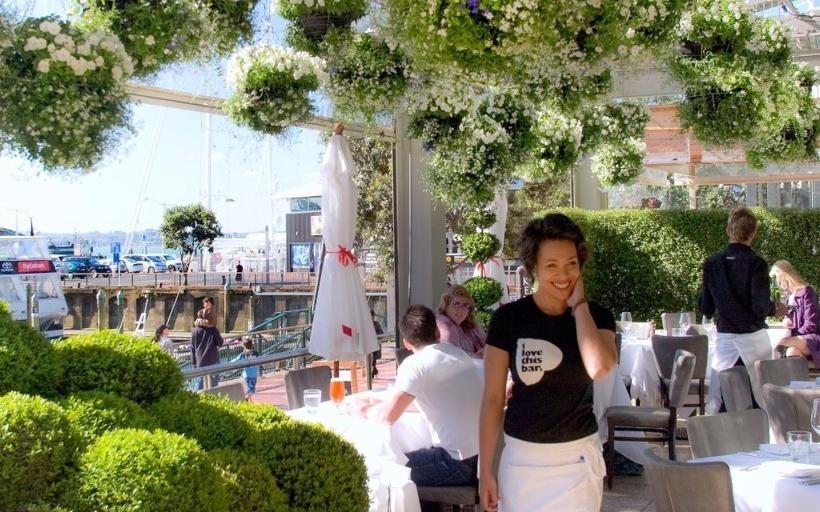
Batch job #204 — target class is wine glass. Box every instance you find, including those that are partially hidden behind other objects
[329,378,346,415]
[680,313,692,336]
[702,314,715,339]
[620,311,633,342]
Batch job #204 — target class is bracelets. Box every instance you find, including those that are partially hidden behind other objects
[571,298,588,317]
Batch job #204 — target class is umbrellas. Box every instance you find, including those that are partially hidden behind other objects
[473,191,510,313]
[305,122,380,379]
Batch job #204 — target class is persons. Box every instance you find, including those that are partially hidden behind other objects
[152,324,176,359]
[193,295,218,328]
[769,259,818,360]
[697,206,772,415]
[234,260,243,283]
[433,286,488,358]
[357,305,484,487]
[190,309,224,392]
[369,309,383,380]
[479,215,618,512]
[229,337,264,403]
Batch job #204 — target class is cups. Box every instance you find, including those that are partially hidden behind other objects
[787,430,812,464]
[672,327,681,337]
[810,398,820,438]
[636,323,650,340]
[304,388,322,415]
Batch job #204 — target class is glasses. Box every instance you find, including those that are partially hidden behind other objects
[452,300,469,310]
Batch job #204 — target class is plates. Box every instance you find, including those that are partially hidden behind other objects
[759,440,820,456]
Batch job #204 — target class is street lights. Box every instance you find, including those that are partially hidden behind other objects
[203,191,234,272]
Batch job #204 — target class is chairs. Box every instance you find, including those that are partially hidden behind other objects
[640,449,735,511]
[719,367,756,413]
[651,333,708,416]
[604,349,696,490]
[662,312,696,335]
[685,408,770,458]
[616,319,668,407]
[416,406,506,512]
[395,346,414,365]
[199,381,247,404]
[754,357,810,409]
[284,366,331,410]
[763,382,819,442]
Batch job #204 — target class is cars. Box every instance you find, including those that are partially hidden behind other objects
[18,254,184,279]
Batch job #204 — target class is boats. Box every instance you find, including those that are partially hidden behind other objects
[169,337,264,361]
[0,217,93,255]
